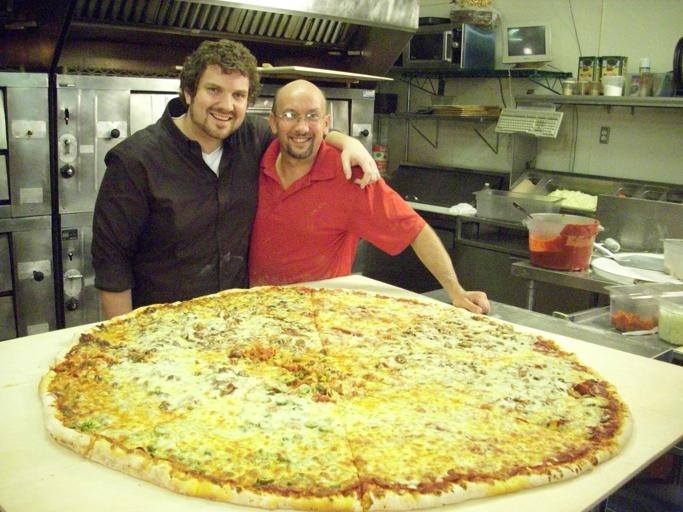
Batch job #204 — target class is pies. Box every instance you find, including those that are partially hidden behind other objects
[39,285,634,511]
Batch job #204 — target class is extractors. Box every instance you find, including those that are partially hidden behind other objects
[2,0,419,82]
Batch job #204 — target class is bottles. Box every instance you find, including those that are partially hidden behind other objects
[563,56,650,96]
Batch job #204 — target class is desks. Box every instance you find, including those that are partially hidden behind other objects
[421,259,683,512]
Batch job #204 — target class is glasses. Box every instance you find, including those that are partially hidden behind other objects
[273,111,323,121]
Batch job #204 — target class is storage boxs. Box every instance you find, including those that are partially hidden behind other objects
[471,189,566,223]
[659,238,683,280]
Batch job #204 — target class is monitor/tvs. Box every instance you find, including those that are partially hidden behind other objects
[503,22,551,68]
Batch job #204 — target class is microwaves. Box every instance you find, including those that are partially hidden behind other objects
[403,22,496,72]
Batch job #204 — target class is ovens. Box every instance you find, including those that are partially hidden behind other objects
[0,72,376,343]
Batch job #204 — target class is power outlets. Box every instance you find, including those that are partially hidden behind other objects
[599,126,610,144]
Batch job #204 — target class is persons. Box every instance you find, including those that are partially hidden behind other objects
[90,38,382,319]
[247,79,490,316]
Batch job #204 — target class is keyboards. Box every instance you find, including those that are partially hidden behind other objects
[494,108,565,139]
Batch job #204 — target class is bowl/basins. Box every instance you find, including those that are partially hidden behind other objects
[417,17,451,25]
